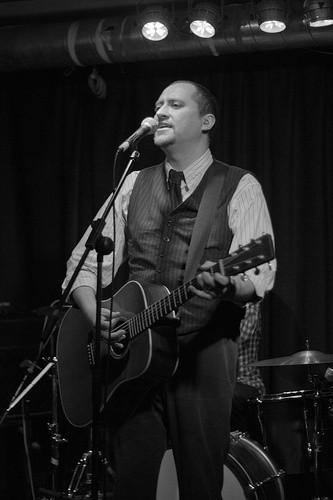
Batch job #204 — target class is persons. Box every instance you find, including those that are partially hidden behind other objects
[235,301,265,402]
[61,81,278,500]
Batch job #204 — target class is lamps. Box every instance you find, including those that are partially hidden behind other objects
[256,1,287,35]
[135,2,171,44]
[302,0,333,30]
[185,2,224,41]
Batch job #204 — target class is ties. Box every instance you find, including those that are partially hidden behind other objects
[168,169,184,212]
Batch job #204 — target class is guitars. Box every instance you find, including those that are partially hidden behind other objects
[54,231,276,430]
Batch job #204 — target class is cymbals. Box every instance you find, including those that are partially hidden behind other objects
[238,349,333,367]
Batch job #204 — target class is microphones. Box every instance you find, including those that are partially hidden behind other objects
[118,117,158,153]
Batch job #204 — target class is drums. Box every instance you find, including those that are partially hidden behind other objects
[246,380,333,481]
[156,431,285,500]
